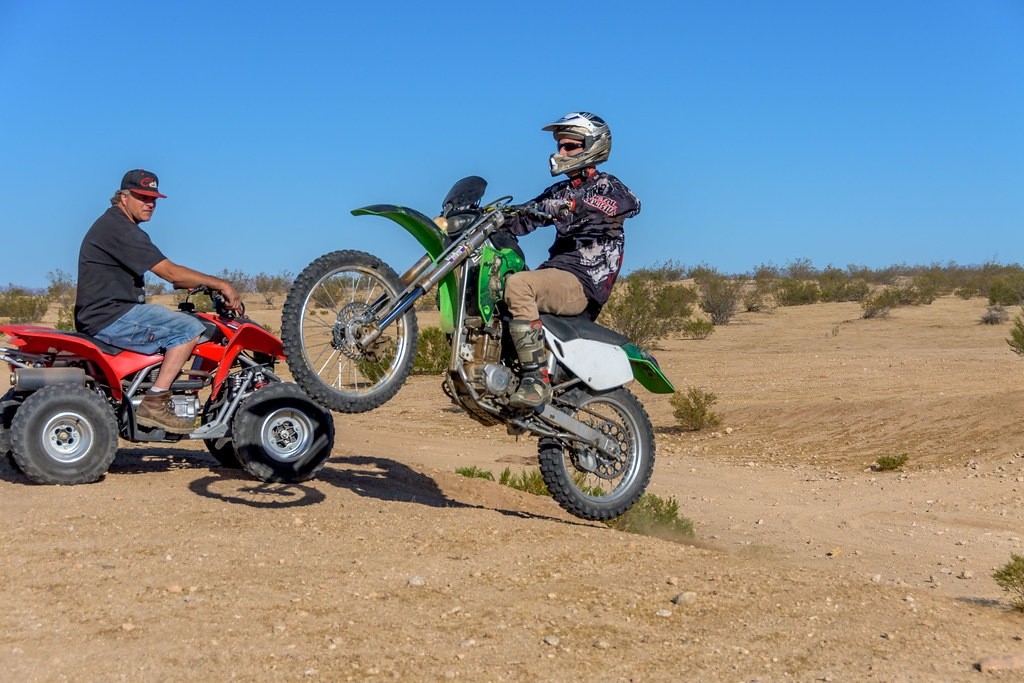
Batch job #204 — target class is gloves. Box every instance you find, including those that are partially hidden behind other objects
[537,199,571,221]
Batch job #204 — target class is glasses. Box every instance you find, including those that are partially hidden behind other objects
[556,142,583,152]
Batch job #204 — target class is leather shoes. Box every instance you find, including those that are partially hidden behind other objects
[136,389,196,434]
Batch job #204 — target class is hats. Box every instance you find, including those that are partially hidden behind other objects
[120,169,168,198]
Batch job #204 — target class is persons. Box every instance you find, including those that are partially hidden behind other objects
[73,169,242,434]
[472,111,641,409]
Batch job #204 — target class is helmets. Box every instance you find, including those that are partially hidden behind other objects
[541,111,612,176]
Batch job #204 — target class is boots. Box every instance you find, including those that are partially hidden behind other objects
[510,318,554,406]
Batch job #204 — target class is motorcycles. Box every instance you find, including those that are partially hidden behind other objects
[0,279,338,487]
[282,175,679,519]
